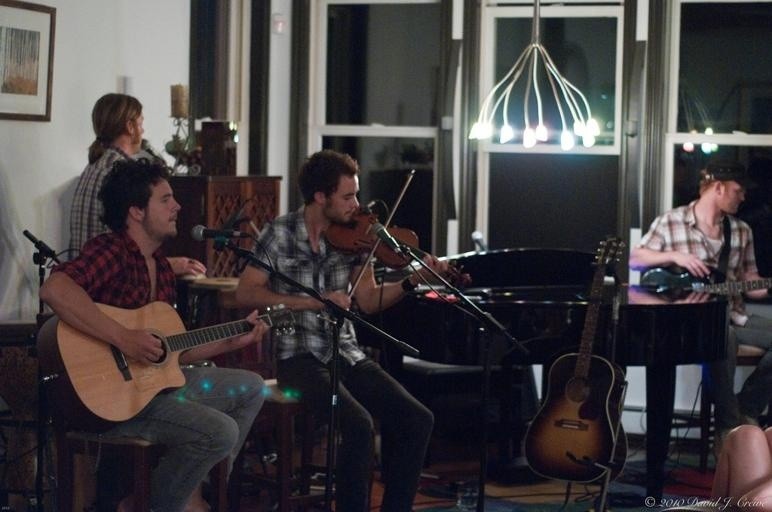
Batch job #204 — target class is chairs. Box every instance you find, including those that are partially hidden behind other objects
[242,306,342,511]
[34,314,228,512]
[698,344,765,473]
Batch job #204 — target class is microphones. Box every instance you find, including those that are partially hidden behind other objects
[373,222,405,257]
[191,225,248,242]
[141,139,163,161]
[610,290,623,322]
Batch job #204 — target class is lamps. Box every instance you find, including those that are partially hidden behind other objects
[165,83,203,174]
[468,0,601,151]
[678,74,719,155]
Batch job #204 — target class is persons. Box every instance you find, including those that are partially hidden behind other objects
[628,161,771,424]
[39,158,271,510]
[68,93,206,276]
[711,425,771,509]
[235,150,435,511]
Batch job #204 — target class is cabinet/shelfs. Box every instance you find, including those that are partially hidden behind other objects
[167,173,283,277]
[368,165,433,252]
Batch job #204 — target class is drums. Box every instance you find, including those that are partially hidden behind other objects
[177,273,238,362]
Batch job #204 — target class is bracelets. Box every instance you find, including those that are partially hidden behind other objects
[401,277,418,291]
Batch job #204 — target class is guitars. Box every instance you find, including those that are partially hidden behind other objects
[640,267,772,299]
[525,236,626,486]
[37,301,295,422]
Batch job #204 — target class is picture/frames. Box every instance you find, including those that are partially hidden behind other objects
[0,0,56,122]
[733,82,772,179]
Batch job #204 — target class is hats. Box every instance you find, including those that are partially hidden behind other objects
[706,159,760,192]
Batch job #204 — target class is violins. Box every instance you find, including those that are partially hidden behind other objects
[326,204,471,287]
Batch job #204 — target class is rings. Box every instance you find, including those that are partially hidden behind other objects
[188,259,195,264]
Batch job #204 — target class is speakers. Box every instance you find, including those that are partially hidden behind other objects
[380,363,539,486]
